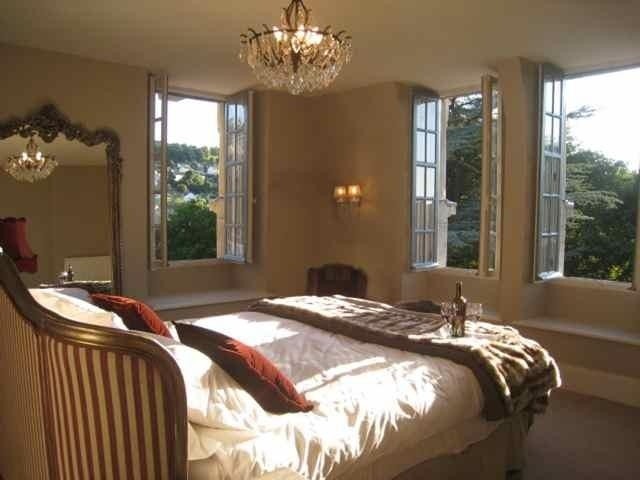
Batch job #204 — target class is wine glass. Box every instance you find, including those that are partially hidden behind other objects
[441,301,483,338]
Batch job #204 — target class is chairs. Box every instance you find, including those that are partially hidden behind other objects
[309,265,368,298]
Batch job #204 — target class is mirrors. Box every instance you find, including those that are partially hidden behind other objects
[0,114,124,298]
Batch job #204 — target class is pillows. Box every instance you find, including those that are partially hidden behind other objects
[91,290,314,415]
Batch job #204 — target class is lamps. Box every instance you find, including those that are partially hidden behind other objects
[2,133,59,186]
[235,0,353,97]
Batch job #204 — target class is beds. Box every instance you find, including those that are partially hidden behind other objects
[0,252,530,480]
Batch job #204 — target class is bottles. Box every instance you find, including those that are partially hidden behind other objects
[67,263,75,281]
[449,278,466,337]
